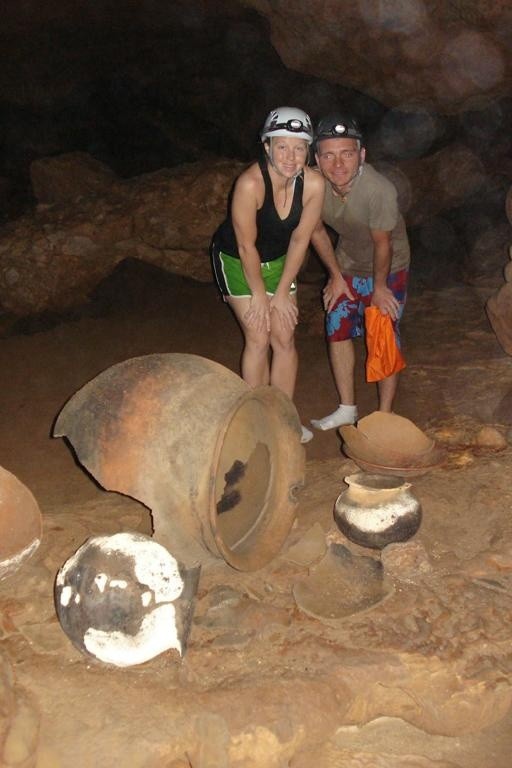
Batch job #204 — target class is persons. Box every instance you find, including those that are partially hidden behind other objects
[308,107,411,432]
[207,104,326,445]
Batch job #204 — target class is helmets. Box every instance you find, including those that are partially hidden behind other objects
[261,108,364,145]
